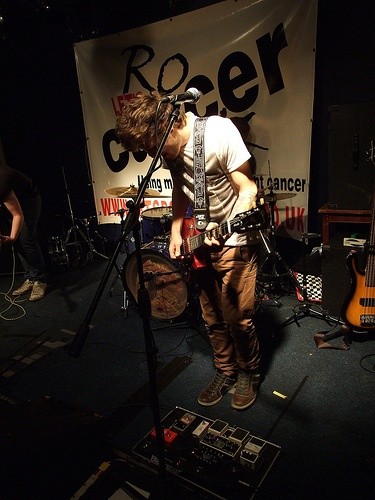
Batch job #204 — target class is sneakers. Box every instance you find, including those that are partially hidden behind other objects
[197,368,236,405]
[231,372,260,410]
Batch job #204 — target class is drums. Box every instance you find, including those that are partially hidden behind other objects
[121,231,202,320]
[141,206,174,244]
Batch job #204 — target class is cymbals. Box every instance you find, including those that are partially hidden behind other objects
[257,193,297,202]
[257,182,278,193]
[104,187,160,197]
[253,174,270,176]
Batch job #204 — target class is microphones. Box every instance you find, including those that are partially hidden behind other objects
[160,87,200,105]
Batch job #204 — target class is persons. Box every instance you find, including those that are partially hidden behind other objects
[0,163,49,301]
[115,92,264,409]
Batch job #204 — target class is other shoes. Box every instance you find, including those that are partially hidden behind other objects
[29,281,46,301]
[12,279,33,295]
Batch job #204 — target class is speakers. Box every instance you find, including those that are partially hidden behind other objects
[322,245,363,314]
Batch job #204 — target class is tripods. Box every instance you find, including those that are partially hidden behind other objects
[258,203,301,291]
[281,232,346,326]
[54,162,108,265]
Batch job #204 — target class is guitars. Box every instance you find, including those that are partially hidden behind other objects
[340,199,375,329]
[177,198,271,272]
